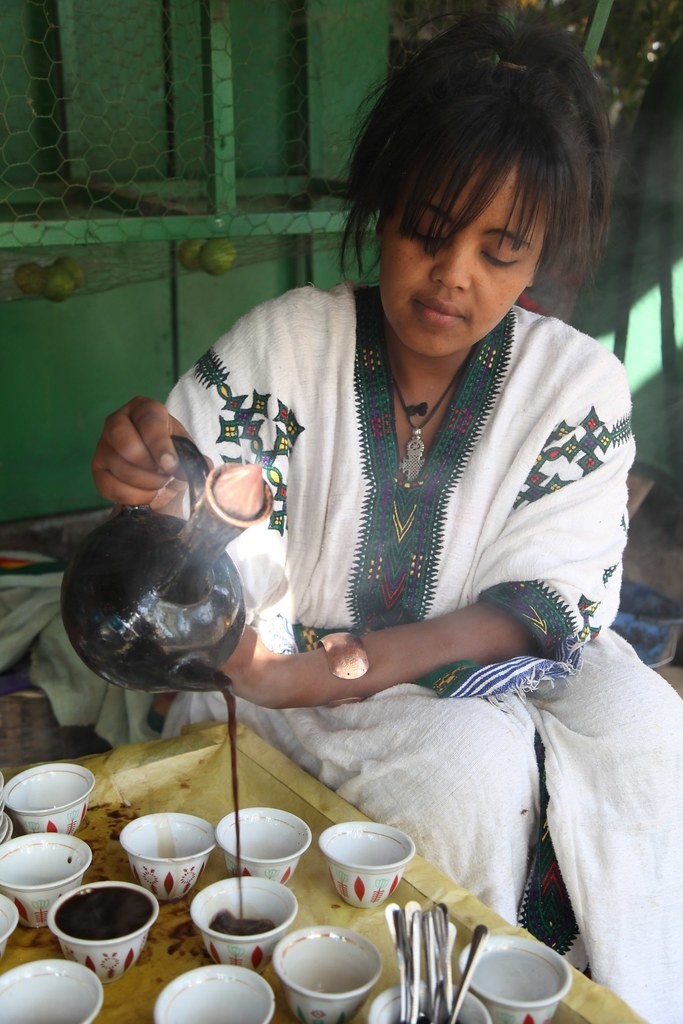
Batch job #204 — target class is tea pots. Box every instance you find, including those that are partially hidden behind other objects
[62,436,274,694]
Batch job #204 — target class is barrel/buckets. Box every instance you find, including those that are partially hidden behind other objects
[609,579,683,670]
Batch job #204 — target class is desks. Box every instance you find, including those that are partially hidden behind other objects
[0,718,647,1024]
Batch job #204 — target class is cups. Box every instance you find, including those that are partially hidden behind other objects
[319,822,416,908]
[154,964,275,1024]
[0,959,104,1024]
[3,763,95,835]
[368,979,493,1024]
[47,881,159,984]
[0,833,92,927]
[0,894,19,963]
[119,813,217,900]
[190,876,298,974]
[271,925,383,1024]
[460,935,573,1024]
[215,807,312,885]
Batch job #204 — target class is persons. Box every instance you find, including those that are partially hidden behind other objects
[91,24,682,1023]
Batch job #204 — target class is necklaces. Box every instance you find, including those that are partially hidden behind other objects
[385,343,479,481]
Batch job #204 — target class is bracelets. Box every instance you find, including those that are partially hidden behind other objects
[316,632,369,708]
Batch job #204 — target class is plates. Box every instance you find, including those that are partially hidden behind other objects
[0,771,13,844]
